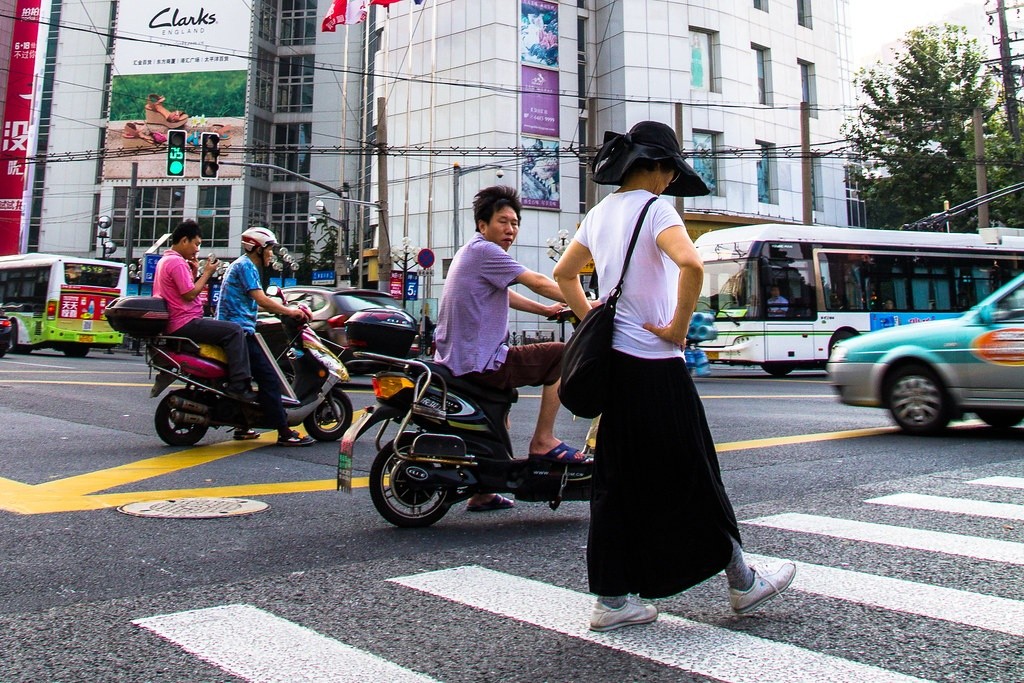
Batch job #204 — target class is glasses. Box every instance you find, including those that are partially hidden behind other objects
[670,168,681,184]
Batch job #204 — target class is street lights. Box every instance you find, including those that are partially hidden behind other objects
[129,255,143,352]
[271,249,299,288]
[309,196,391,295]
[195,253,225,319]
[547,229,575,343]
[388,237,421,308]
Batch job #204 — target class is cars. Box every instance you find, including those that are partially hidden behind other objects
[0,316,13,359]
[827,272,1024,436]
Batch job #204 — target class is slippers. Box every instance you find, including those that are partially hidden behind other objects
[466,492,515,511]
[528,442,589,464]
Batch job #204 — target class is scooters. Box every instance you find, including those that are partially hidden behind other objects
[338,291,601,528]
[104,296,353,447]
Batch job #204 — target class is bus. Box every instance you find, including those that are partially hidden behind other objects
[692,182,1023,376]
[0,253,128,358]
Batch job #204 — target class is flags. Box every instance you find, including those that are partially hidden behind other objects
[321,0,401,33]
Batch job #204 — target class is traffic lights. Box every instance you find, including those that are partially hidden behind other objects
[201,132,220,178]
[167,130,186,177]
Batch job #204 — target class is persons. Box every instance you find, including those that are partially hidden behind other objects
[152,219,258,402]
[216,227,316,445]
[419,309,433,356]
[885,298,894,309]
[435,184,589,512]
[768,286,788,317]
[552,121,796,631]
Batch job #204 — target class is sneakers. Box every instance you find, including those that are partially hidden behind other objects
[275,429,318,447]
[728,562,797,614]
[589,593,659,632]
[226,384,258,403]
[233,429,260,440]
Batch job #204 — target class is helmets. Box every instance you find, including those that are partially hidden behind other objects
[241,227,279,252]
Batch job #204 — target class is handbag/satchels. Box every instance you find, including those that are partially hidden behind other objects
[556,286,624,418]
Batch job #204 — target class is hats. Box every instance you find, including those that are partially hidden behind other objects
[590,120,712,197]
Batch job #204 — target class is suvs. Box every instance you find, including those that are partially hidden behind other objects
[257,287,408,364]
